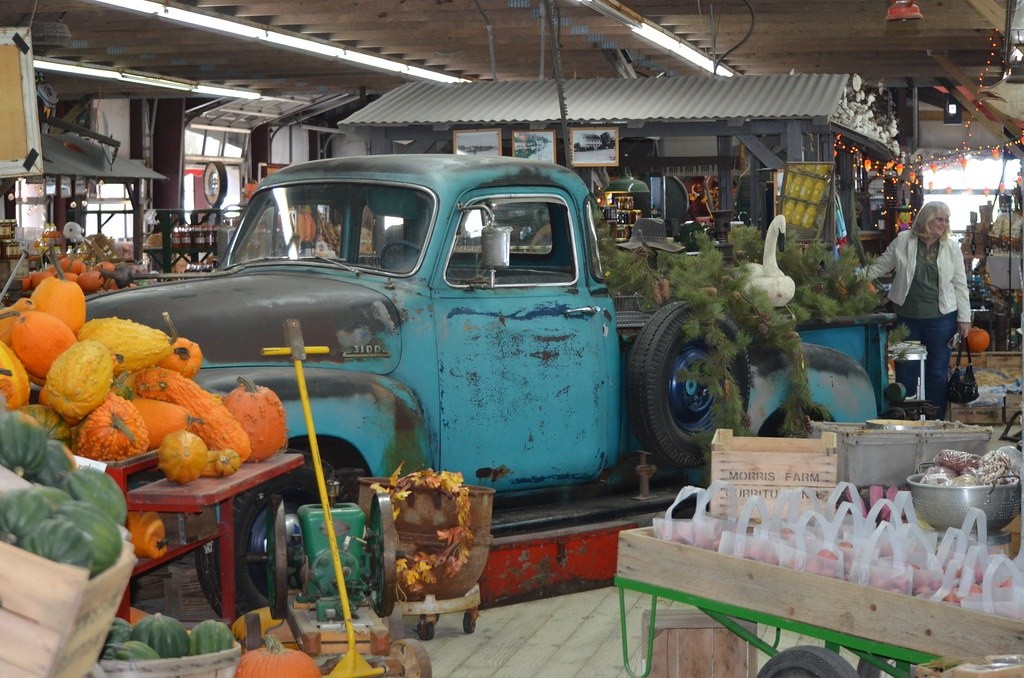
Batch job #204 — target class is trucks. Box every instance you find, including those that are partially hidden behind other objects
[27,154,910,621]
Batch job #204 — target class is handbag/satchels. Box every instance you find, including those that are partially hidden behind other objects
[652,481,1023,620]
[948,335,979,402]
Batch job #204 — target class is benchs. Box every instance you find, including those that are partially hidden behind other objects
[32,0,476,100]
[446,263,572,283]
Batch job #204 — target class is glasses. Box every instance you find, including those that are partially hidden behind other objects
[936,217,950,224]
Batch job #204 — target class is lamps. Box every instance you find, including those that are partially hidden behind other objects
[601,154,651,195]
[884,0,924,23]
[576,0,742,79]
[943,96,962,125]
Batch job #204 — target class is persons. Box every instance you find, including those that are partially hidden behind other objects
[855,200,973,419]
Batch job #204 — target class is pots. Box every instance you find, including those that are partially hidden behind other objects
[357,476,496,601]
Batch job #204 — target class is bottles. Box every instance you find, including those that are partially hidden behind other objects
[0,219,95,279]
[172,225,220,248]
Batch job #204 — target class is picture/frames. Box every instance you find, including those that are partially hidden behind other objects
[512,130,557,165]
[452,128,502,156]
[0,27,44,178]
[570,126,619,167]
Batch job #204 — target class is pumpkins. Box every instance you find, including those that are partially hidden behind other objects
[0,259,322,677]
[959,328,993,351]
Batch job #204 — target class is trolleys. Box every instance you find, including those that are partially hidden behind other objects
[614,526,1023,678]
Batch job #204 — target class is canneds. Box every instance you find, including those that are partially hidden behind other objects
[601,197,641,245]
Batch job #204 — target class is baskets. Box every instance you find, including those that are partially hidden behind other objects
[90,630,241,678]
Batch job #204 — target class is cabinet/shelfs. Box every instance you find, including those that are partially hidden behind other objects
[83,207,219,284]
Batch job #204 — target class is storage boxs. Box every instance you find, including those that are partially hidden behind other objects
[0,463,136,678]
[711,429,838,527]
